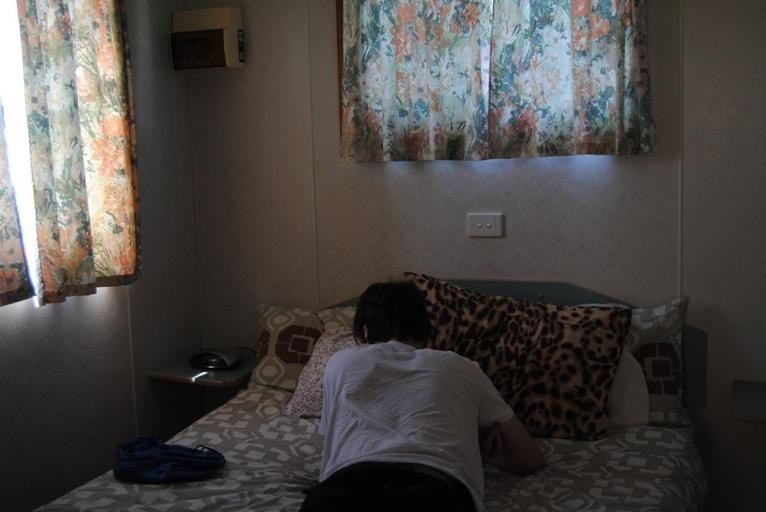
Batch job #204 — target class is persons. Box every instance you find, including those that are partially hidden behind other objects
[297,277,541,511]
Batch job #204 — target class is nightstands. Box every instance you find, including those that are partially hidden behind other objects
[145,363,255,421]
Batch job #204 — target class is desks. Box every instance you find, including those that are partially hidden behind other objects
[732,380,766,424]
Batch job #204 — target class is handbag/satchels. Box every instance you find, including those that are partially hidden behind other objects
[114,434,226,484]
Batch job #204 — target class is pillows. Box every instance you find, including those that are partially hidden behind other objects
[252,302,358,392]
[606,351,650,428]
[281,328,357,417]
[622,296,694,429]
[388,271,632,441]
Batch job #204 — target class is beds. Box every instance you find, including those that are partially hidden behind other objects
[32,280,707,512]
